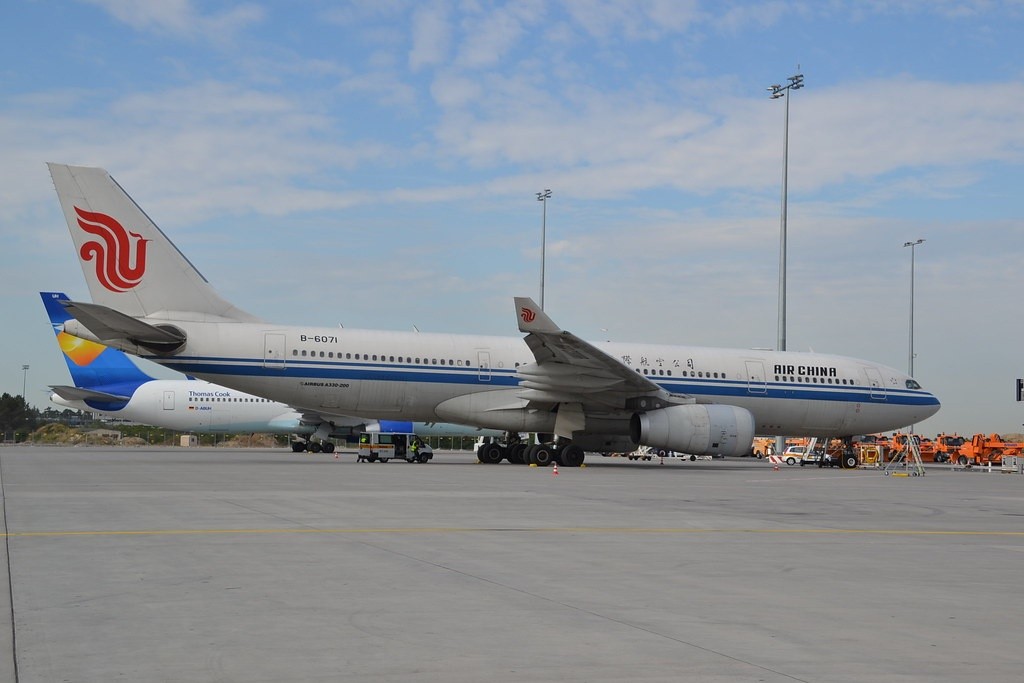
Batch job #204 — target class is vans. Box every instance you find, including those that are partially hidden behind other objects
[358,431,435,463]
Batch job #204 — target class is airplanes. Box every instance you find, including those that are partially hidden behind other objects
[47,159,939,467]
[39,290,530,454]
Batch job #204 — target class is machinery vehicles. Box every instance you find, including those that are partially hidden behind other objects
[602,432,1023,467]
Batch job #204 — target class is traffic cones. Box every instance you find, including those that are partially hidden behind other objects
[335,451,339,458]
[552,462,558,474]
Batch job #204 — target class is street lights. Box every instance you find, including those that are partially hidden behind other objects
[903,239,926,378]
[22,364,29,399]
[535,188,554,314]
[768,71,805,352]
